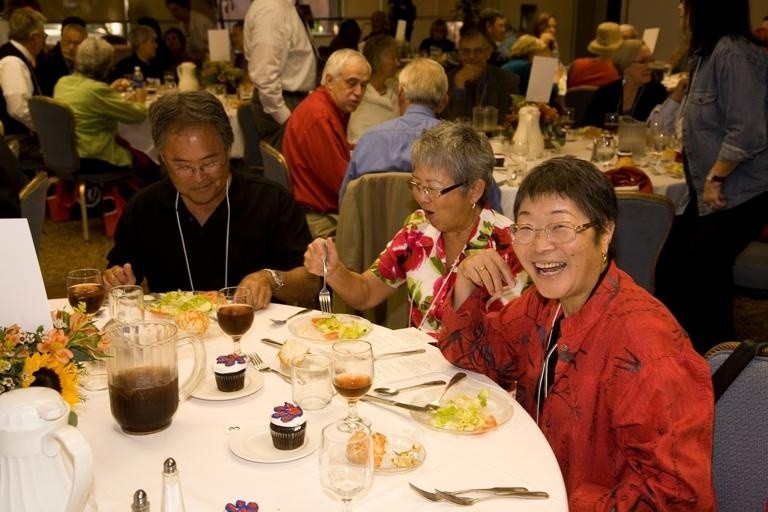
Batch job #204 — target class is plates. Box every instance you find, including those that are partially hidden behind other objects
[182,363,271,401]
[408,379,514,437]
[287,312,375,343]
[146,289,225,316]
[226,423,326,464]
[344,429,429,476]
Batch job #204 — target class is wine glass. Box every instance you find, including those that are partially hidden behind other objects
[216,286,255,356]
[67,267,105,324]
[329,338,375,435]
[319,419,376,510]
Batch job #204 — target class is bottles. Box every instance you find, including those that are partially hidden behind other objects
[161,457,187,512]
[132,488,149,511]
[470,99,686,183]
[309,16,344,37]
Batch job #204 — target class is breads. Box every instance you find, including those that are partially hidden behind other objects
[347,429,386,467]
[280,343,310,367]
[615,157,636,169]
[175,309,210,337]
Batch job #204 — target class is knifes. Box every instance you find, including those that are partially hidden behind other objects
[360,393,441,413]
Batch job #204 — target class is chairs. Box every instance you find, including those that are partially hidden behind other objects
[0,45,767,510]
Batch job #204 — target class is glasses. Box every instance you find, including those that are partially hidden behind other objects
[408,180,463,201]
[170,151,230,180]
[507,220,595,244]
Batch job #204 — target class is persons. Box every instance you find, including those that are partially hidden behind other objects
[0,0,768,339]
[439,156,715,511]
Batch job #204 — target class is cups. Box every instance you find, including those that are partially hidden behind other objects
[109,285,147,322]
[289,353,334,413]
[266,422,307,450]
[78,345,115,395]
[213,369,245,392]
[104,58,255,110]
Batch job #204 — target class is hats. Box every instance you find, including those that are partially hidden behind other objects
[588,22,625,55]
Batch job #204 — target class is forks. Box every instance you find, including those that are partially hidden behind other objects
[433,486,549,508]
[269,307,317,325]
[406,480,530,500]
[317,241,334,315]
[246,350,302,387]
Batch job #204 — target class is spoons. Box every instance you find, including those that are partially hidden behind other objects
[424,372,469,414]
[369,380,446,396]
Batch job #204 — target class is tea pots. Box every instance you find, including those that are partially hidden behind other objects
[0,386,97,512]
[105,319,208,436]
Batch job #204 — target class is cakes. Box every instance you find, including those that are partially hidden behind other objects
[224,499,260,512]
[213,352,247,392]
[269,401,306,450]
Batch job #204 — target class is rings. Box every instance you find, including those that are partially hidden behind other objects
[476,265,485,272]
